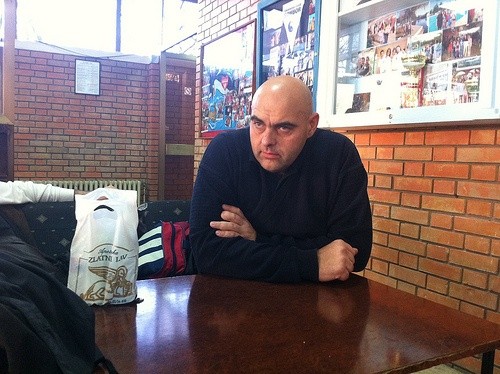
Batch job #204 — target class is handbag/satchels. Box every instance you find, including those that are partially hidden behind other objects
[67,186,139,305]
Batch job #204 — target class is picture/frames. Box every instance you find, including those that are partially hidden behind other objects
[316,0,499,129]
[199,18,257,139]
[256,0,320,128]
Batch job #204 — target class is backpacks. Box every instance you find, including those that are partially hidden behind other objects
[137,221,195,279]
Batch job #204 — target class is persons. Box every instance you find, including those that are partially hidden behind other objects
[189,74,374,283]
[0,180,117,374]
[201,76,251,131]
[356,7,479,104]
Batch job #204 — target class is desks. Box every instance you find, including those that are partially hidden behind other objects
[91,274,500,374]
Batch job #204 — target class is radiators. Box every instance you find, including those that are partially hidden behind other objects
[33,178,146,210]
